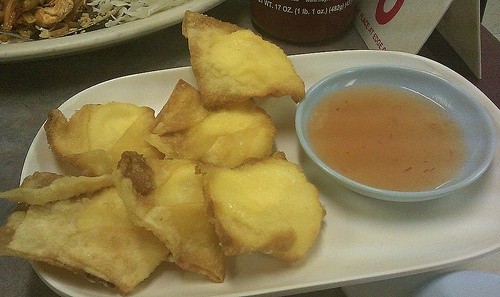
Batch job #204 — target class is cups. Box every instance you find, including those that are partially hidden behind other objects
[250,0,355,43]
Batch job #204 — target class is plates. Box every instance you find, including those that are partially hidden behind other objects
[20,49,499,297]
[0,0,226,63]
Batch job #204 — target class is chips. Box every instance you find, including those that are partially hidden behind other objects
[0,10,328,297]
